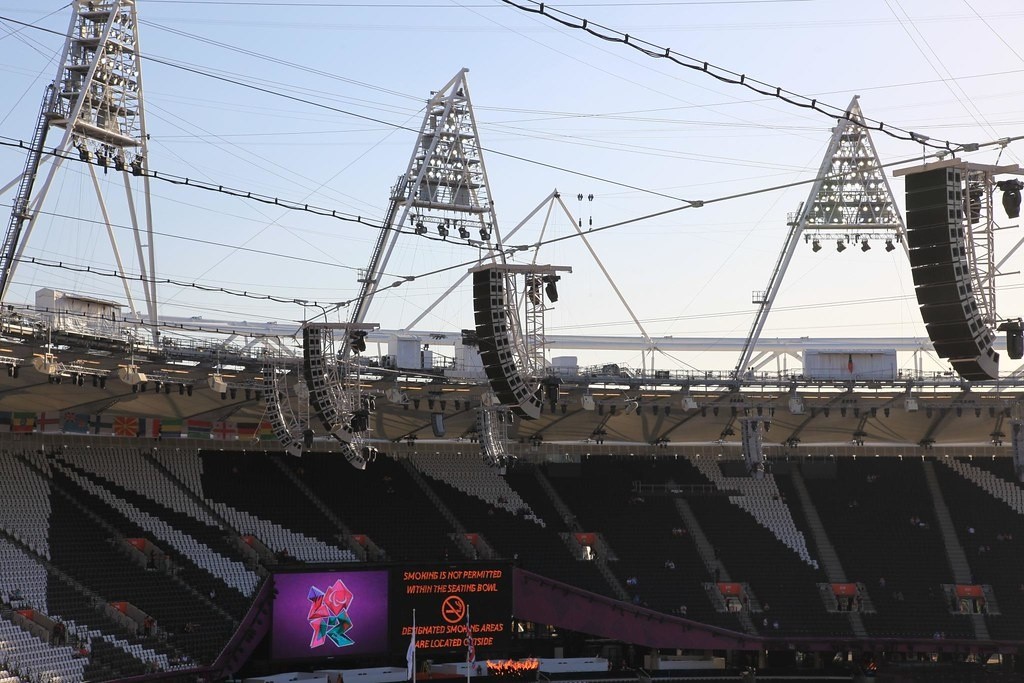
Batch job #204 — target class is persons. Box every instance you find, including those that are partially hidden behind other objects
[144,615,151,637]
[52,622,66,647]
